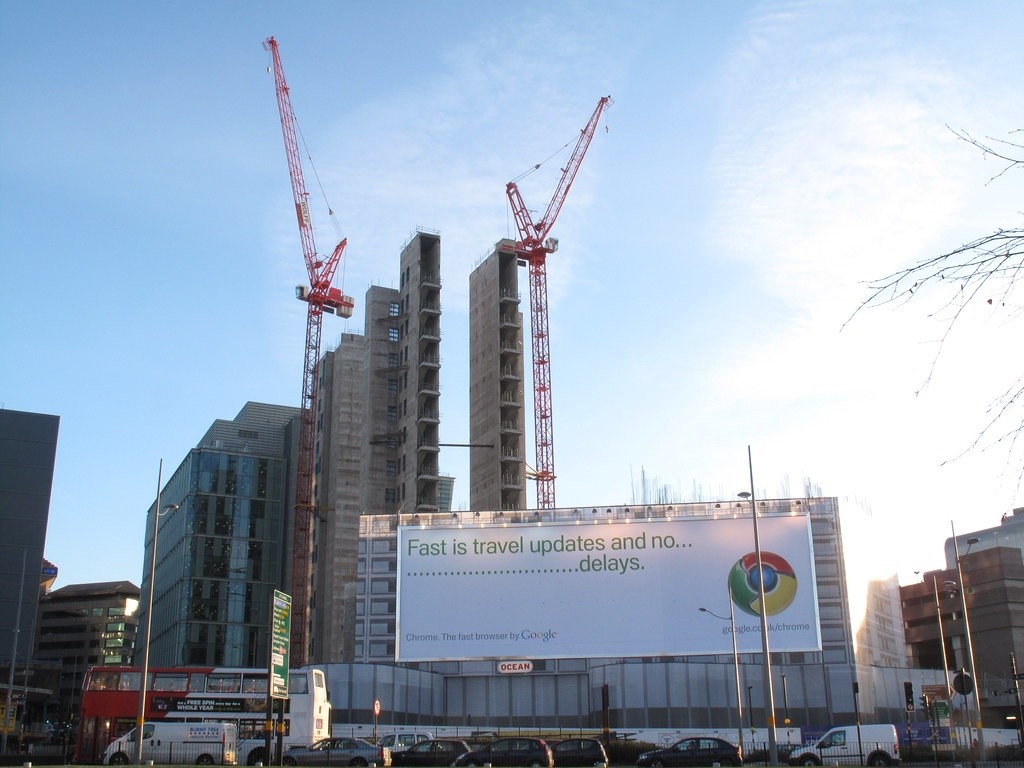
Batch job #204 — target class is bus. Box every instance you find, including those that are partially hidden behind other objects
[72,665,329,766]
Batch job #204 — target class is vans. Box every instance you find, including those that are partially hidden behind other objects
[101,722,238,767]
[791,725,900,768]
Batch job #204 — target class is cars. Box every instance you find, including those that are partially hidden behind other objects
[549,737,608,768]
[636,735,743,768]
[451,739,555,768]
[521,740,569,751]
[57,718,81,743]
[282,734,393,767]
[378,732,493,768]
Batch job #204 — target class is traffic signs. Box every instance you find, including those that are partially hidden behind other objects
[992,673,1023,696]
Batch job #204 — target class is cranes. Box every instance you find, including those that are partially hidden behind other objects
[265,35,356,668]
[494,93,612,507]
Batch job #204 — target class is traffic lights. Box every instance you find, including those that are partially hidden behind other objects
[41,719,50,724]
[904,681,915,714]
[920,695,930,722]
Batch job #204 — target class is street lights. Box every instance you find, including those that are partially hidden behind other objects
[958,538,988,764]
[739,492,778,768]
[133,503,180,765]
[747,684,757,751]
[953,670,976,767]
[699,608,744,748]
[1,595,52,755]
[935,581,957,766]
[782,673,791,748]
[853,682,863,766]
